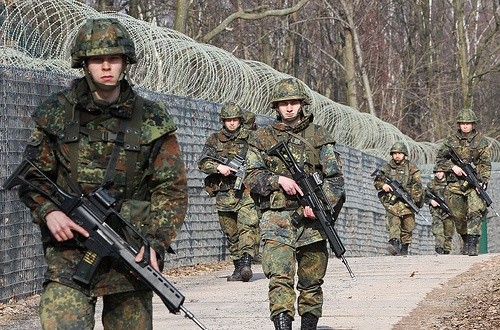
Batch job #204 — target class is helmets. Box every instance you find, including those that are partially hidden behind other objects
[456,109,477,124]
[390,142,408,156]
[220,102,244,119]
[241,111,255,129]
[270,78,312,109]
[69,18,137,67]
[433,165,443,171]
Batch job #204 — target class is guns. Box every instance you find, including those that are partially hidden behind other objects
[424,189,451,214]
[3,157,208,330]
[444,147,500,219]
[369,168,428,222]
[205,148,249,190]
[267,141,356,281]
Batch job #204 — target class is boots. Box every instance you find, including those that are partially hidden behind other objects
[227,259,242,281]
[444,250,449,254]
[400,244,409,255]
[240,252,252,282]
[272,312,293,330]
[301,313,318,330]
[386,238,399,256]
[460,233,468,255]
[435,247,443,253]
[467,234,479,256]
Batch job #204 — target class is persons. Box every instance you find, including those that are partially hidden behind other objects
[374,141,423,255]
[436,109,492,255]
[19,18,188,330]
[243,78,345,330]
[197,102,258,282]
[423,167,455,255]
